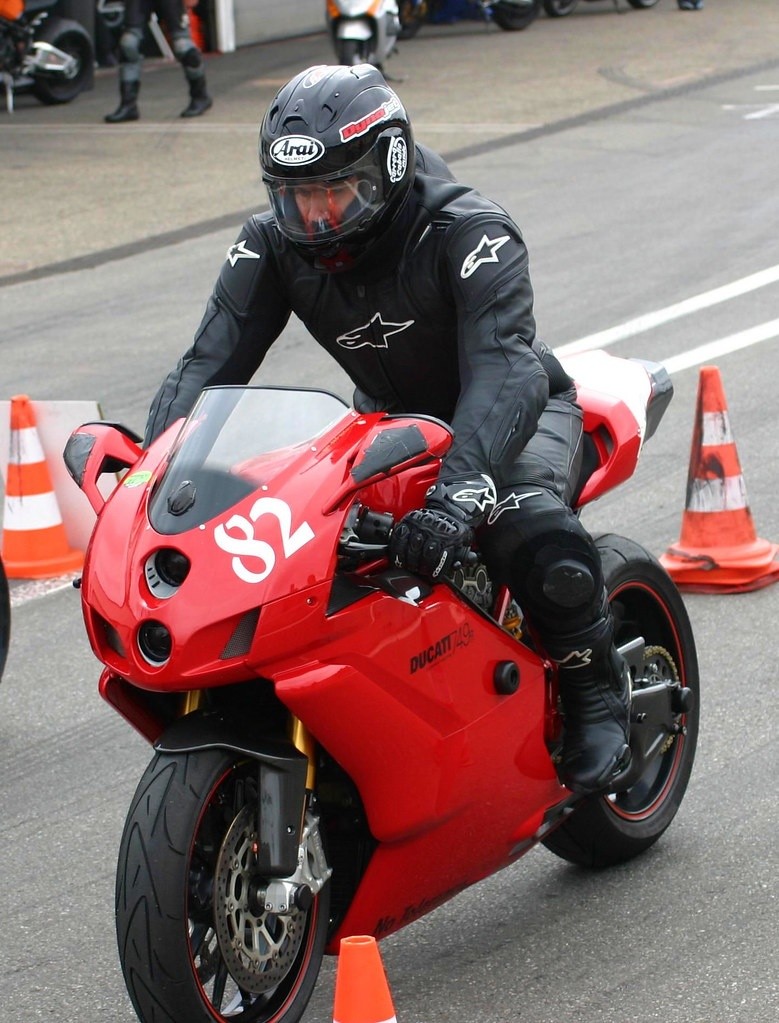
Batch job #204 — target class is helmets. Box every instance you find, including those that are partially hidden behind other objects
[262,64,417,270]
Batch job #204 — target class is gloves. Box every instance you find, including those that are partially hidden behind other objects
[389,477,498,580]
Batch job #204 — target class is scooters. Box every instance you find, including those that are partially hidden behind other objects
[323,0,407,88]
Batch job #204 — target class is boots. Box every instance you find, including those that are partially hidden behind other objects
[104,82,139,122]
[546,588,632,794]
[178,77,212,118]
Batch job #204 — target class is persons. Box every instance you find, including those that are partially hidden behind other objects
[95,1,212,123]
[133,61,634,797]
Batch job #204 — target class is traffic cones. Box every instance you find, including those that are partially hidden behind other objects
[0,395,89,577]
[330,934,401,1023]
[656,364,779,596]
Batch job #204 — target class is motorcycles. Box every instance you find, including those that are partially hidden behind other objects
[61,349,703,1023]
[0,0,94,118]
